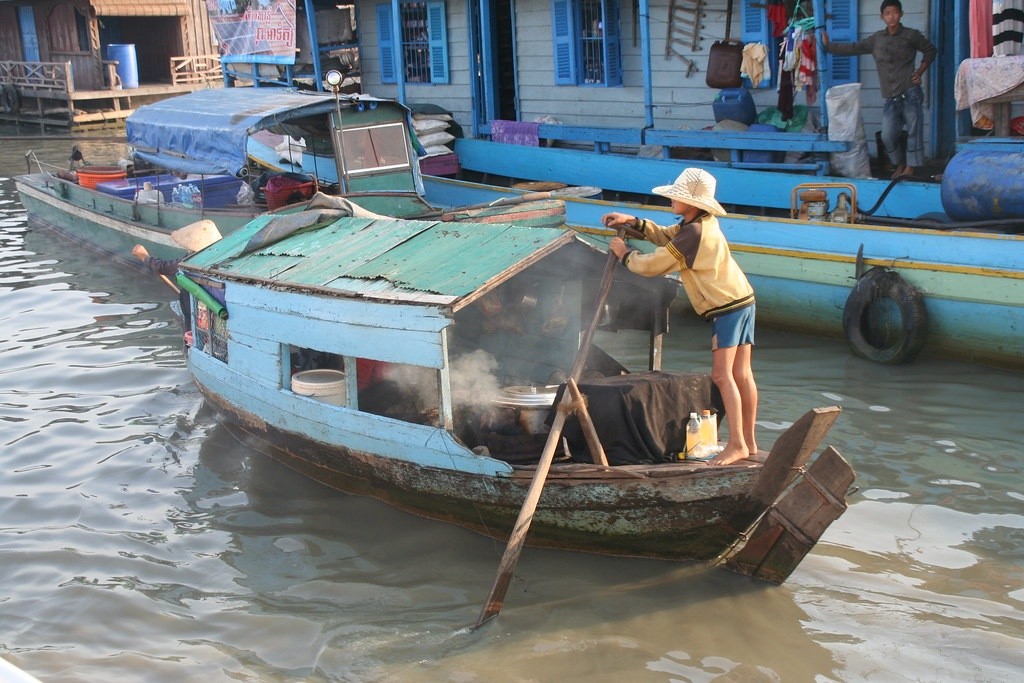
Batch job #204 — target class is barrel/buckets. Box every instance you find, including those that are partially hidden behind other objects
[941,150,1024,221]
[77,166,128,190]
[713,88,757,126]
[261,181,313,210]
[107,44,138,88]
[291,370,346,406]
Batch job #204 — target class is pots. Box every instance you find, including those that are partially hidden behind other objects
[492,385,560,434]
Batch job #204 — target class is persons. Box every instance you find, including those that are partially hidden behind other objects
[355,359,400,416]
[602,168,758,466]
[822,0,937,183]
[132,220,222,349]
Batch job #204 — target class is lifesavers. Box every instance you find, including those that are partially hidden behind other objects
[842,269,926,364]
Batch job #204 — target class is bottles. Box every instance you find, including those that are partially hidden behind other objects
[686,413,700,457]
[706,39,745,88]
[137,182,164,202]
[172,184,202,209]
[586,63,603,84]
[701,410,717,446]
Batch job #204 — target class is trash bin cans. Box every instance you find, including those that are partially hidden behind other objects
[876,130,908,167]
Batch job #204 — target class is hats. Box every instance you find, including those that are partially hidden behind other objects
[171,219,223,252]
[652,168,726,216]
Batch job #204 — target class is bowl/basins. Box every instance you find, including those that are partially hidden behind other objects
[186,331,192,343]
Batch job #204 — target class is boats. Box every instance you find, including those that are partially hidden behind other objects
[131,194,860,585]
[412,133,1024,374]
[12,86,427,280]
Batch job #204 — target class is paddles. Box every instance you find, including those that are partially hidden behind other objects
[470,217,647,627]
